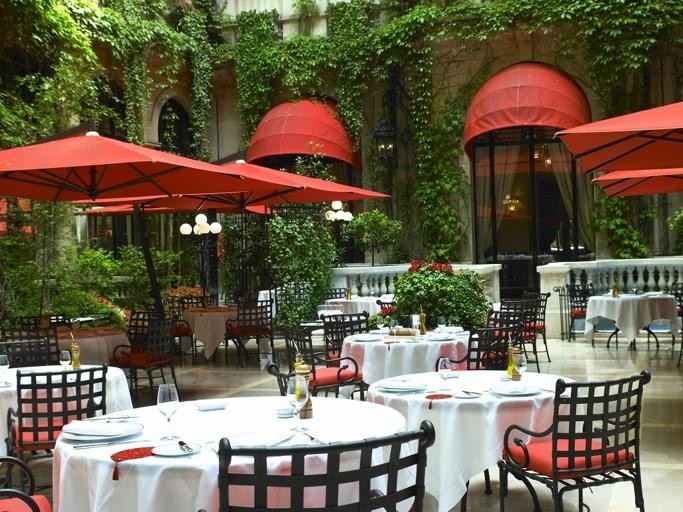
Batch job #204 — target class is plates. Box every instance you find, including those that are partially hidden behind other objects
[353,325,464,343]
[149,443,201,458]
[374,377,540,400]
[60,421,144,442]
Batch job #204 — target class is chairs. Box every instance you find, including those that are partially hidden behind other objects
[4,363,108,495]
[113,308,159,388]
[0,456,52,512]
[1,280,682,369]
[497,369,651,511]
[217,419,436,512]
[122,316,181,392]
[264,363,317,394]
[280,325,363,398]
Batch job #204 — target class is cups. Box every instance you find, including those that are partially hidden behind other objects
[436,317,446,328]
[513,352,526,376]
[0,354,10,373]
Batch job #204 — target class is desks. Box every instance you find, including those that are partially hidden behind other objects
[340,329,481,384]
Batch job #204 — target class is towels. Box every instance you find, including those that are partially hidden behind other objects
[504,381,527,392]
[62,417,129,436]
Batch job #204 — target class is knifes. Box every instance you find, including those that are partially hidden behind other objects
[72,441,136,449]
[178,441,192,452]
[301,432,326,446]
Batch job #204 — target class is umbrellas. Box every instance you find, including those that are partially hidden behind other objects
[592,168,683,198]
[554,101,683,174]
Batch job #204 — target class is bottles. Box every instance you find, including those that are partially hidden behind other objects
[346,287,351,301]
[70,340,82,370]
[612,284,617,298]
[410,304,427,336]
[507,345,523,380]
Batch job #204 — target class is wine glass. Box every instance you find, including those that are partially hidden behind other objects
[59,351,70,372]
[376,316,385,337]
[437,357,452,392]
[155,383,181,441]
[315,304,344,322]
[390,319,400,343]
[69,315,100,341]
[370,286,375,298]
[286,377,310,434]
[607,284,612,298]
[631,283,637,297]
